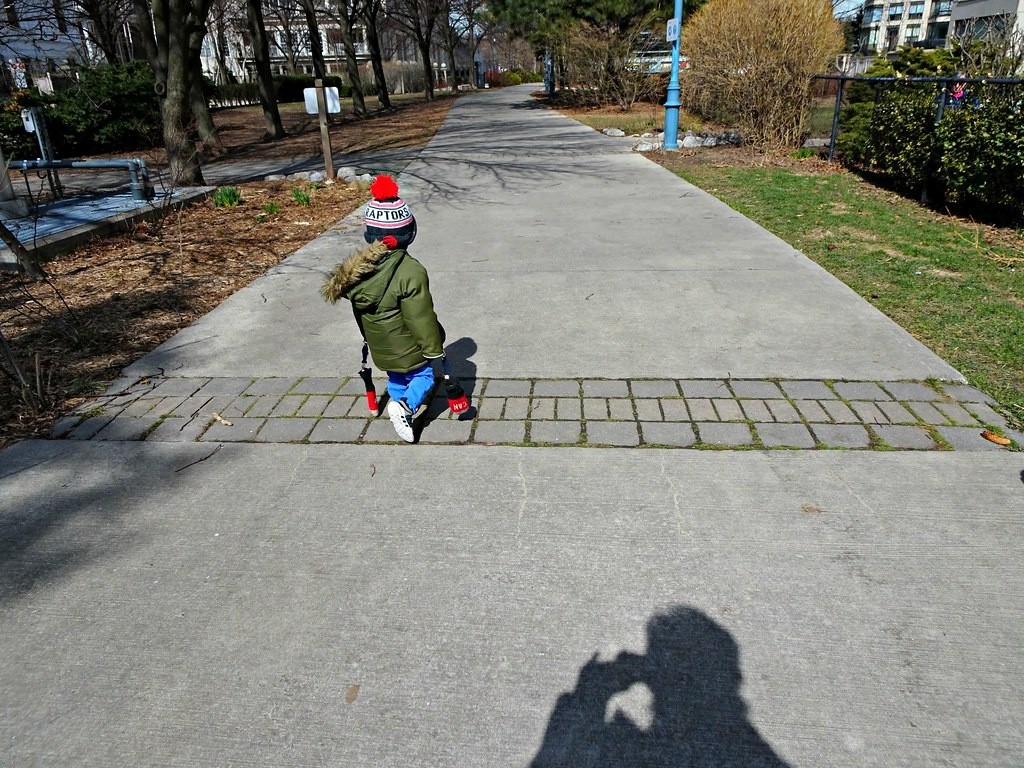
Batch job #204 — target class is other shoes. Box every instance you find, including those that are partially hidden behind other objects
[388,397,415,443]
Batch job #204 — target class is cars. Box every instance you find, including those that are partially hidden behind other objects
[623,50,691,75]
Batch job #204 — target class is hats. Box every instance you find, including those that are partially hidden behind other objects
[364,175,418,248]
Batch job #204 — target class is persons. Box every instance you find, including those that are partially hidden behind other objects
[318,175,450,443]
